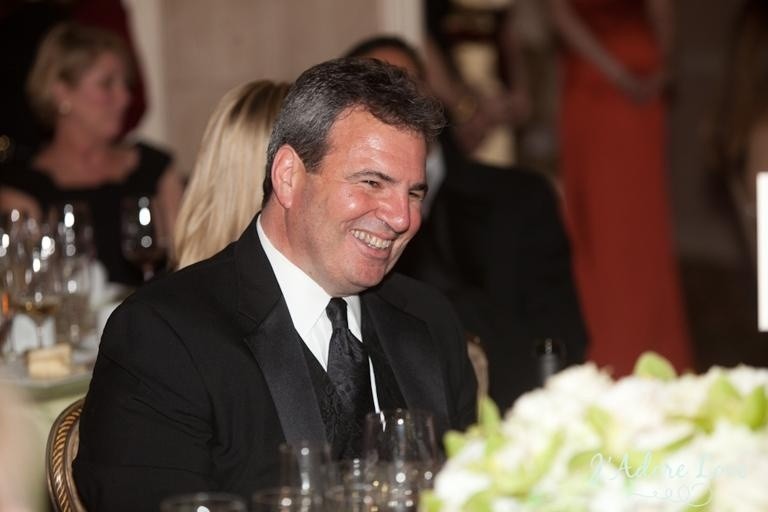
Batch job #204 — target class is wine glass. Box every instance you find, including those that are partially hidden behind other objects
[0,195,171,367]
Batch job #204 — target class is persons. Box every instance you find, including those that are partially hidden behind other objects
[411,1,565,193]
[59,50,480,512]
[342,33,590,421]
[665,0,768,378]
[3,23,185,302]
[169,76,292,279]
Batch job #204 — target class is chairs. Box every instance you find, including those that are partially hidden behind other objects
[44,393,87,512]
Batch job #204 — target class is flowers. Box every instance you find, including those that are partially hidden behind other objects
[414,348,767,512]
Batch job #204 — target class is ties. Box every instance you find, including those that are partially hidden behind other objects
[323,296,386,463]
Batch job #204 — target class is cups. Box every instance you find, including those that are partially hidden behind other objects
[162,408,438,512]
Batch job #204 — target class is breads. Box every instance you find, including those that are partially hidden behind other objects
[25,342,72,378]
